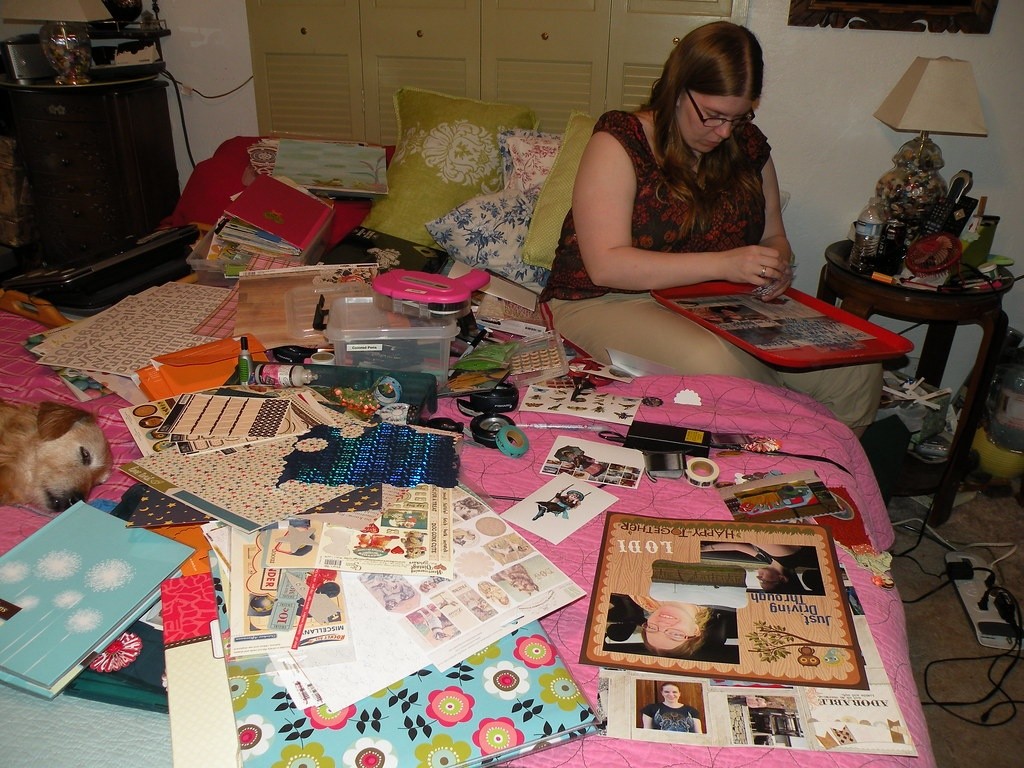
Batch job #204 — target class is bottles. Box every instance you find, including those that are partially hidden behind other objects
[254,363,319,387]
[846,196,885,271]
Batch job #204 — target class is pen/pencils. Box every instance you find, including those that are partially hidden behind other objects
[515,424,607,429]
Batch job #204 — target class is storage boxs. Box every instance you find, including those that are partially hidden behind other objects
[325,294,460,394]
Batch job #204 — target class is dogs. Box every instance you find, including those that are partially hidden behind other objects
[0,398,112,514]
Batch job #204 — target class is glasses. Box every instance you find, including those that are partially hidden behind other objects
[686,88,755,128]
[642,622,696,642]
[759,579,780,585]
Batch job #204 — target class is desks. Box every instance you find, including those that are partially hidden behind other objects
[815,240,1016,527]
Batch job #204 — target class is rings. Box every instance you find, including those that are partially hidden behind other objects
[761,266,766,276]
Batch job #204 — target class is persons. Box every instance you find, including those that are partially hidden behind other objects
[676,302,743,323]
[700,542,807,594]
[640,683,702,733]
[729,697,772,707]
[536,22,883,440]
[629,594,715,658]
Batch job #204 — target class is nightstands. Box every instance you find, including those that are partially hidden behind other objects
[0,80,181,265]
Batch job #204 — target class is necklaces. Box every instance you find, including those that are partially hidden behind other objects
[544,452,639,488]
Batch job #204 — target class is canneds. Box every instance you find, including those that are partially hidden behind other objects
[878,220,907,274]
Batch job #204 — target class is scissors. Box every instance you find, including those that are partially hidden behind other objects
[567,357,614,401]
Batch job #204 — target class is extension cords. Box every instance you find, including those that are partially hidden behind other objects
[944,551,1024,650]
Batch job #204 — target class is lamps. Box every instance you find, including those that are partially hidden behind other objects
[873,57,988,222]
[0,0,113,85]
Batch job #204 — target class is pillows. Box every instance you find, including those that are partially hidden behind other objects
[523,107,601,269]
[360,85,540,252]
[425,125,566,294]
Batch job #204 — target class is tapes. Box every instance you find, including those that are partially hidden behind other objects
[495,425,529,458]
[683,458,721,489]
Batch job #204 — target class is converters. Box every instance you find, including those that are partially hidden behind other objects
[623,420,712,459]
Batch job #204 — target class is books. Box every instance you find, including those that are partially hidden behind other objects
[274,138,387,195]
[160,551,603,768]
[215,173,332,256]
[0,500,196,701]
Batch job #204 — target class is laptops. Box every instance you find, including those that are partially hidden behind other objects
[2,225,199,292]
